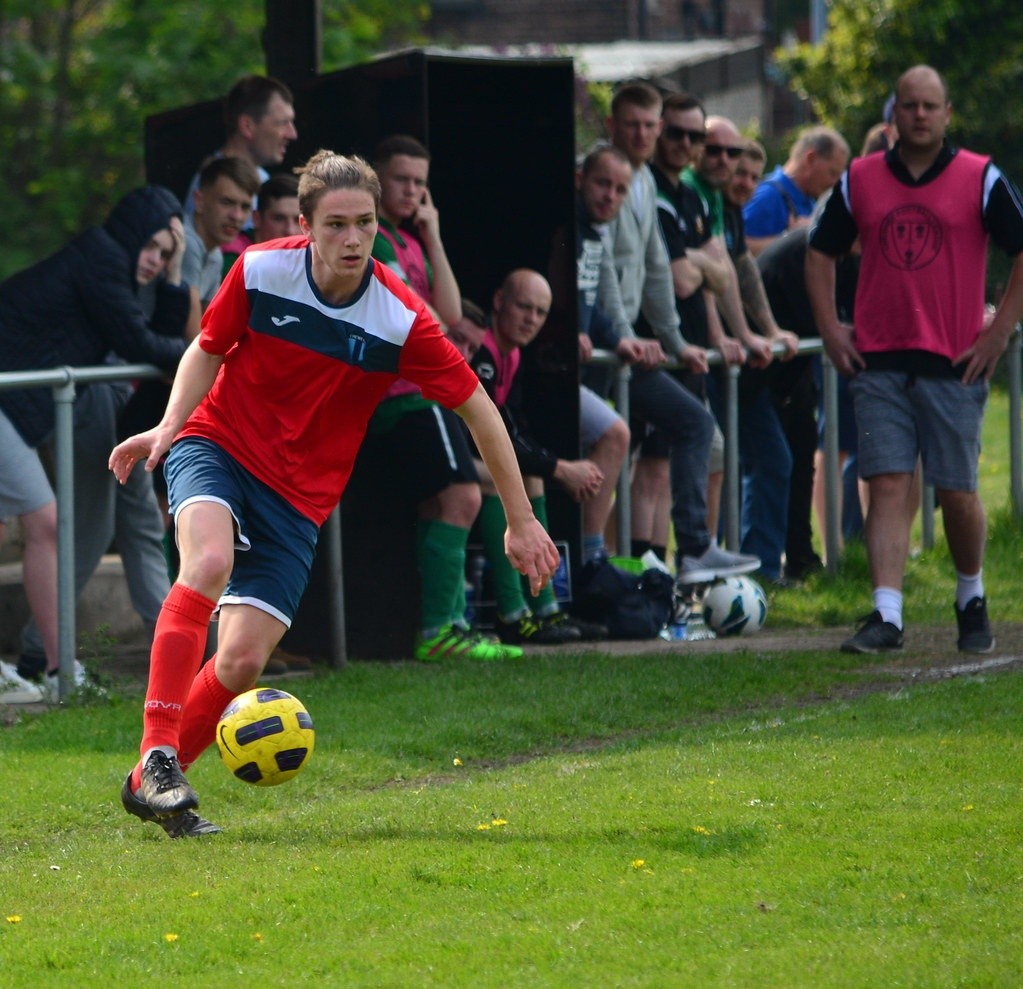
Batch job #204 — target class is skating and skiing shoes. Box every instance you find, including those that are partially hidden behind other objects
[676,544,761,585]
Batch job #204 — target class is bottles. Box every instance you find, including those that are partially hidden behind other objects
[660,619,716,641]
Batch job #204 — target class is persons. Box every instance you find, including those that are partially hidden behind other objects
[805,67,1023,657]
[0,80,945,701]
[109,150,563,837]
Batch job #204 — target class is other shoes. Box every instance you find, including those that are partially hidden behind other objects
[264,647,312,673]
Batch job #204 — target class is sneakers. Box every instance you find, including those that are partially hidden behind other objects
[412,620,523,663]
[0,658,112,705]
[496,609,608,643]
[660,591,706,640]
[953,596,994,654]
[121,750,220,838]
[841,611,904,654]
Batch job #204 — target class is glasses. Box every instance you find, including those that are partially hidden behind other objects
[666,123,705,143]
[706,144,744,159]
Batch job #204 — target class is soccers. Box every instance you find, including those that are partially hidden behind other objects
[702,575,769,637]
[216,687,315,787]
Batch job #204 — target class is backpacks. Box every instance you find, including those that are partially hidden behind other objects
[573,559,673,637]
